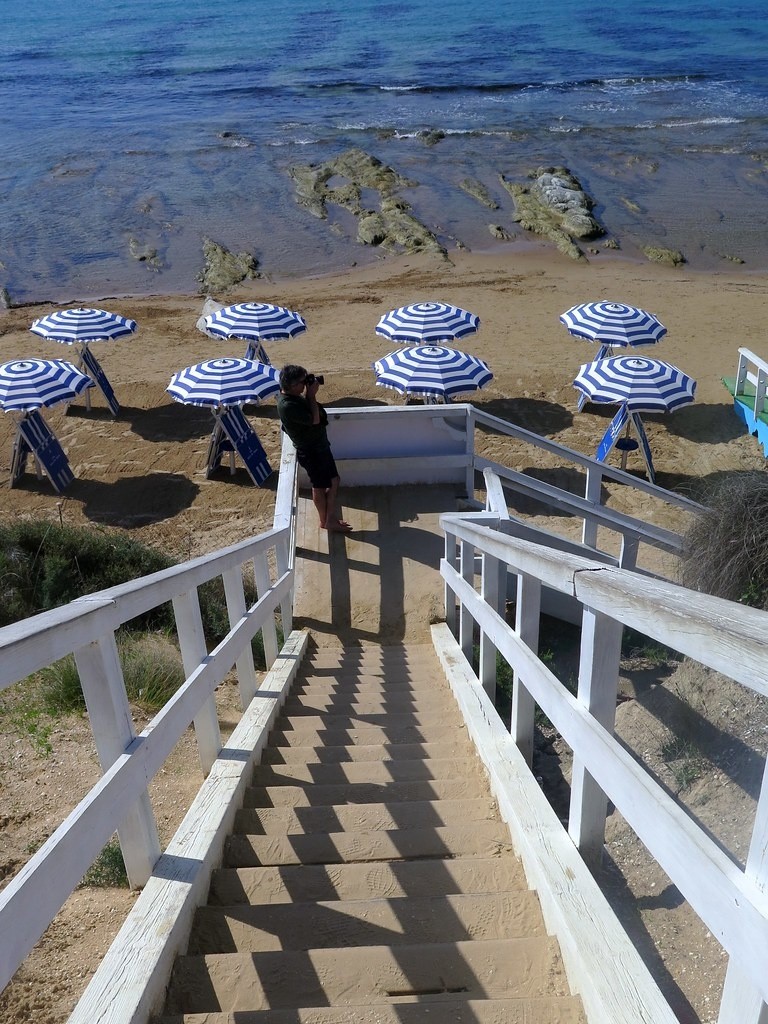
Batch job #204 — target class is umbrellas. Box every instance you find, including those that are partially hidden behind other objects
[375,301,481,345]
[166,358,282,475]
[29,307,139,412]
[372,345,493,405]
[572,355,697,470]
[559,300,667,357]
[0,359,96,480]
[204,301,308,358]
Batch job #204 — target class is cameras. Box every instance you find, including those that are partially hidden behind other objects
[307,373,325,386]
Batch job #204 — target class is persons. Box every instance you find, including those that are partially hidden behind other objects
[277,365,354,532]
